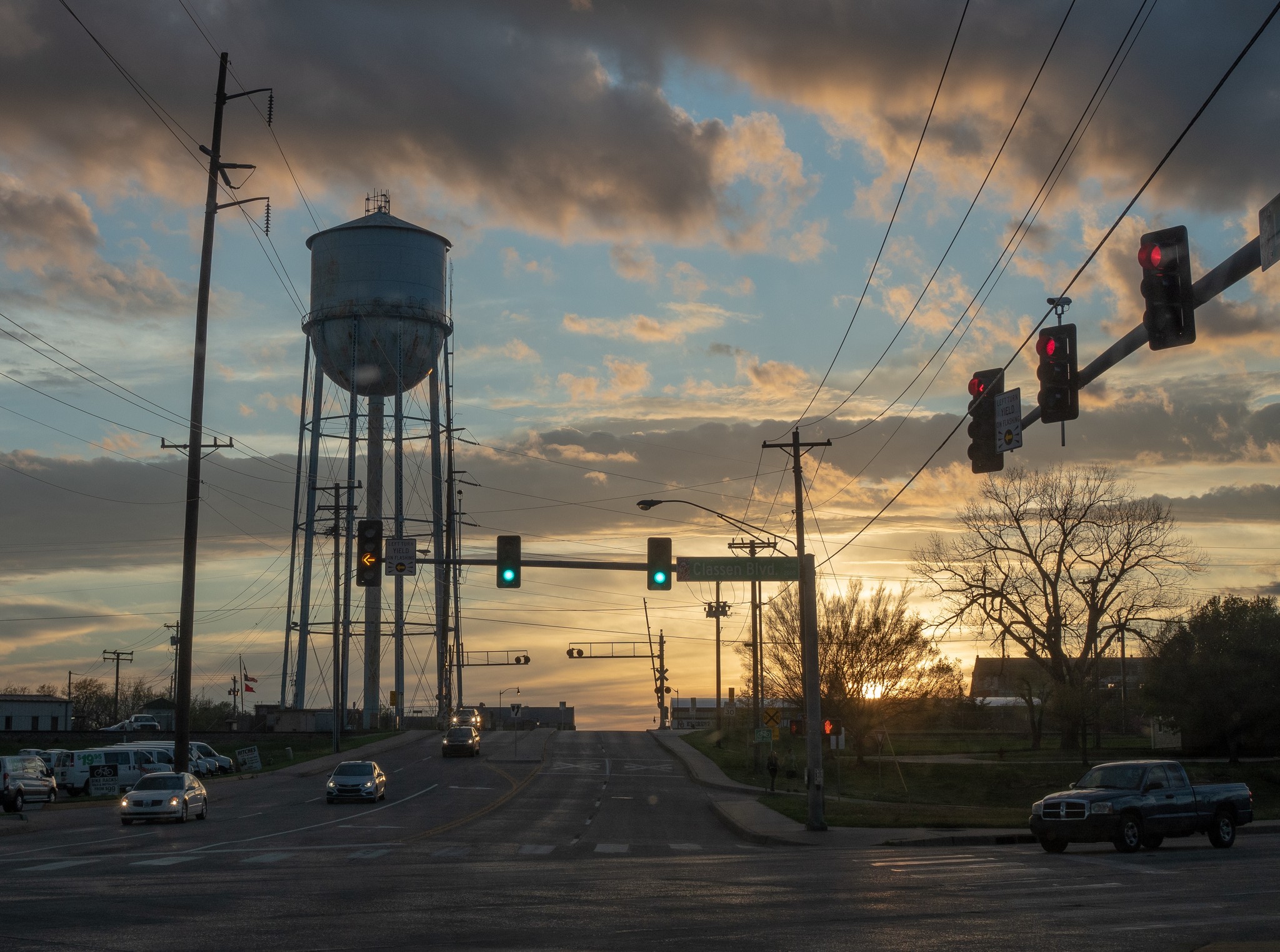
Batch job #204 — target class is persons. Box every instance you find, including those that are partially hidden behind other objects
[766,750,780,792]
[782,748,799,792]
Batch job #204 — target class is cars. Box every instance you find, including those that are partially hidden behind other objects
[120,772,208,825]
[326,761,386,805]
[442,726,480,757]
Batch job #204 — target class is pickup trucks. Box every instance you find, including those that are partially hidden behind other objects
[1028,760,1253,854]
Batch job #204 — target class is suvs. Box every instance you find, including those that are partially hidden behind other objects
[98,714,161,732]
[453,708,481,732]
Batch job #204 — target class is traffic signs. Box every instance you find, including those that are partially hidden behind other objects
[995,387,1023,454]
[385,538,417,576]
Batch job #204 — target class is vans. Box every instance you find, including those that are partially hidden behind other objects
[18,741,233,794]
[0,755,58,813]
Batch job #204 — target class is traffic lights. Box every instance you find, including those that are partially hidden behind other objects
[496,535,522,588]
[967,367,1004,474]
[647,537,671,590]
[822,719,843,736]
[356,520,384,587]
[1138,225,1196,351]
[789,720,805,735]
[1035,323,1079,424]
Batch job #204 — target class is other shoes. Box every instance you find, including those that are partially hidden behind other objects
[770,787,775,791]
[796,789,801,793]
[787,788,791,792]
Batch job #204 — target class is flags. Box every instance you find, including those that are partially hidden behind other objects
[245,683,256,692]
[244,673,258,683]
[242,660,247,675]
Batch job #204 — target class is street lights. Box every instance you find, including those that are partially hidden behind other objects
[653,715,669,729]
[523,717,540,730]
[635,498,807,717]
[500,686,520,727]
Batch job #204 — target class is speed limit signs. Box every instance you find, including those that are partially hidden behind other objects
[723,701,736,717]
